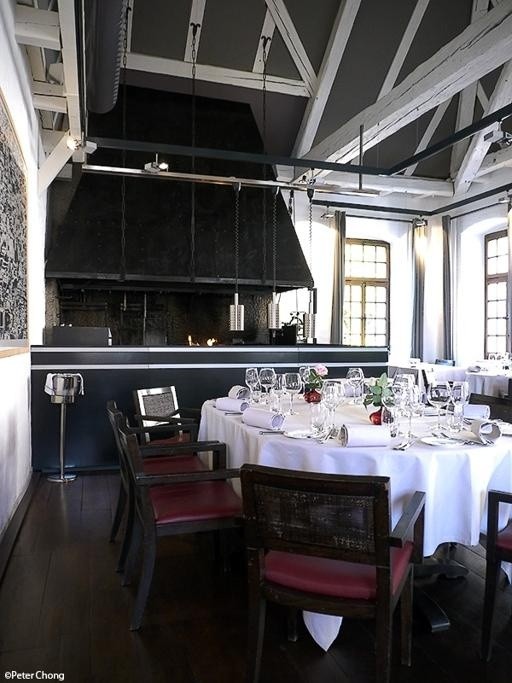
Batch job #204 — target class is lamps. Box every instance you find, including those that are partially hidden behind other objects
[266,186,282,329]
[304,189,316,337]
[484,121,512,146]
[230,180,245,331]
[66,129,98,153]
[143,152,169,173]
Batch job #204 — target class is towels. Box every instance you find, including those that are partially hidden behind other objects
[43,372,85,396]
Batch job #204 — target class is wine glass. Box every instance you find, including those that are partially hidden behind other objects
[244,366,301,416]
[320,380,346,426]
[427,379,470,430]
[383,372,424,447]
[346,365,365,405]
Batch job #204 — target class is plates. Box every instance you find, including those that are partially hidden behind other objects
[482,421,512,437]
[281,424,324,439]
[421,434,466,446]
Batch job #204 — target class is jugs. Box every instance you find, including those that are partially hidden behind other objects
[42,147,318,289]
